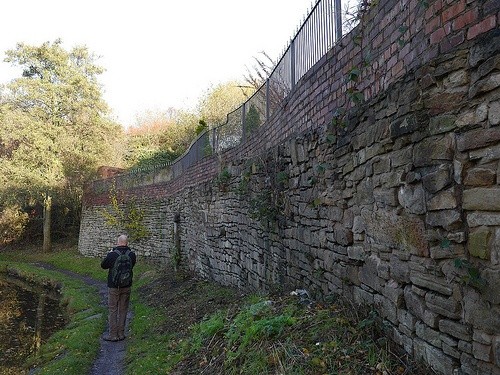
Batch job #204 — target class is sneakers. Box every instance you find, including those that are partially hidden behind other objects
[103,335,118,341]
[119,335,125,340]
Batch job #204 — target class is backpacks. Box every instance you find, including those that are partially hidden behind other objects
[112,249,134,288]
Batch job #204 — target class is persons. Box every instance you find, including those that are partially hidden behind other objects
[101,234,137,342]
